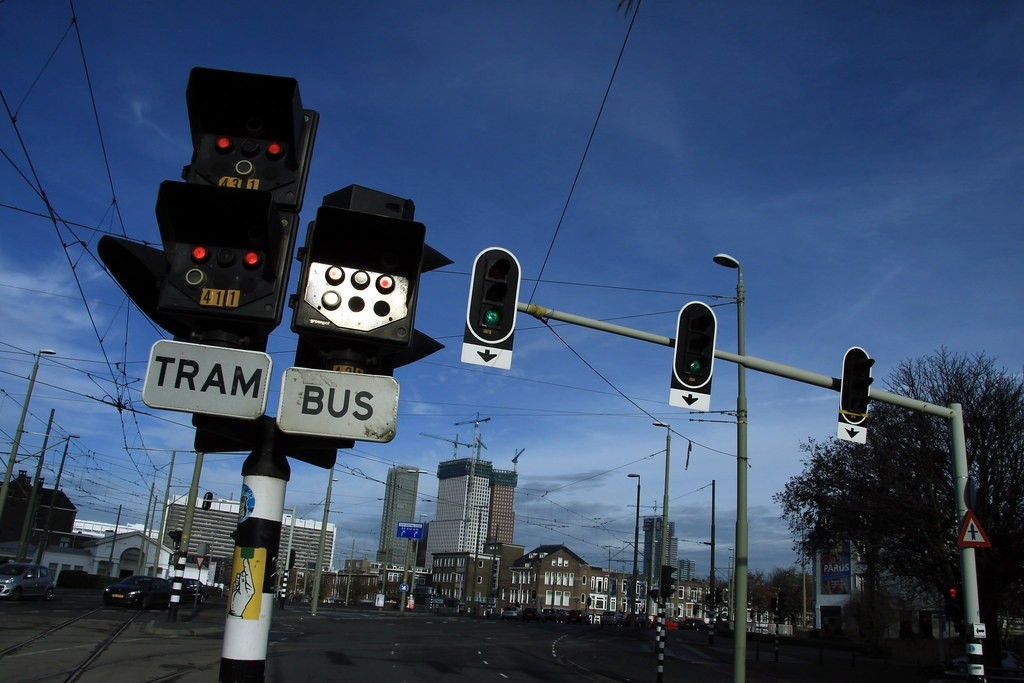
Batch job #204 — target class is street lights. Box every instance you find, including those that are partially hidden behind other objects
[0,349,57,514]
[628,475,641,616]
[653,422,672,610]
[409,514,429,607]
[711,251,749,683]
[37,434,81,565]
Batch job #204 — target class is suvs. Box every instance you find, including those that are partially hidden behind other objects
[501,607,588,624]
[600,612,651,629]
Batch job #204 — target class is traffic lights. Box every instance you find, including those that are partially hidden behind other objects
[673,300,716,390]
[465,247,520,343]
[947,579,963,607]
[839,346,877,425]
[660,564,676,598]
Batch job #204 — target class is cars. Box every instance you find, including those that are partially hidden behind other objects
[104,576,209,610]
[0,563,54,601]
[683,619,707,630]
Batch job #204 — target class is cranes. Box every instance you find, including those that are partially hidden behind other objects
[418,432,473,460]
[476,433,487,462]
[512,448,526,471]
[454,412,490,552]
